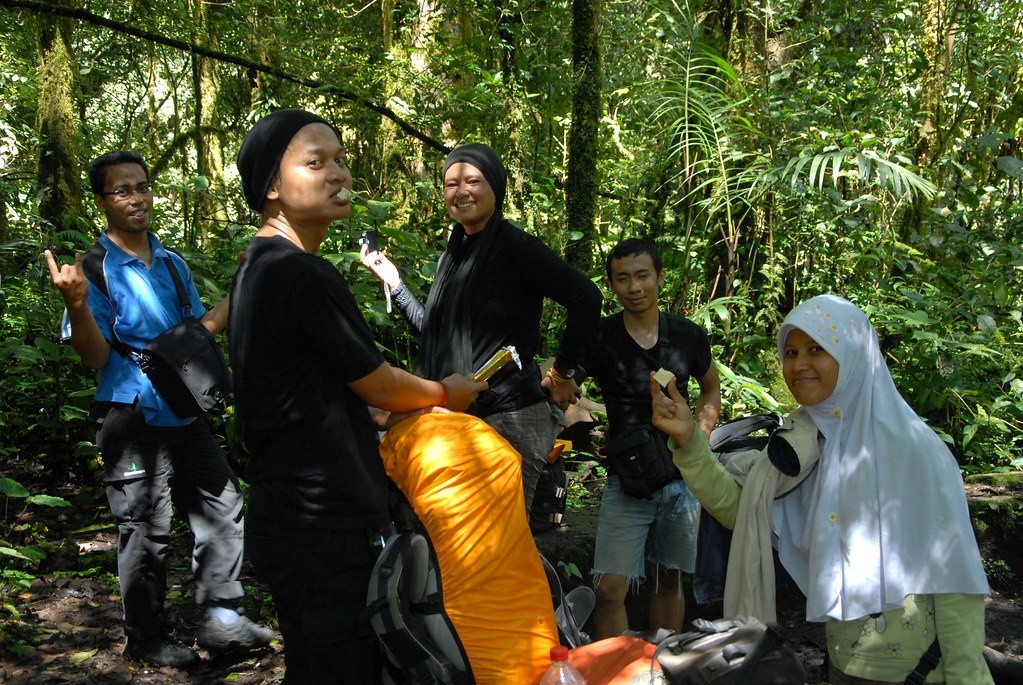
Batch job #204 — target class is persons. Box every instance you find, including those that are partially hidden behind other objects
[539,238,722,640]
[359,141,603,527]
[45,152,273,664]
[650,293,996,685]
[228,110,491,684]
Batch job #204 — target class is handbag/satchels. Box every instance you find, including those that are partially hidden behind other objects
[110,258,233,420]
[607,424,676,501]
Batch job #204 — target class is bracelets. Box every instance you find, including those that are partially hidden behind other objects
[545,366,576,389]
[438,382,447,408]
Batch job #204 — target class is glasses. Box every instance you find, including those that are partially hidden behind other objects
[104,184,153,201]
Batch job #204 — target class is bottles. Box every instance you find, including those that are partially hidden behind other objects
[540,645,586,685]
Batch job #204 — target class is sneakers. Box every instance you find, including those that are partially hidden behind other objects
[197,615,275,648]
[122,633,201,668]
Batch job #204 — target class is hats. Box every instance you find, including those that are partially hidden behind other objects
[236,107,343,213]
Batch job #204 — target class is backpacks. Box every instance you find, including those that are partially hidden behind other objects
[710,413,784,453]
